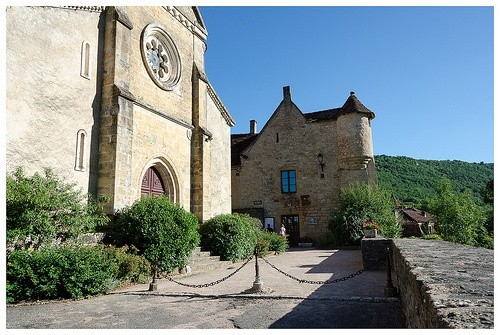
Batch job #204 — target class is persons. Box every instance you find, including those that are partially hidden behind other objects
[279,224,287,237]
[265,223,273,233]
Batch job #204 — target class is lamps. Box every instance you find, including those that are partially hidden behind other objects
[317,153,325,172]
[303,194,310,200]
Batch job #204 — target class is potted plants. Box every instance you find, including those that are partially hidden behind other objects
[363,222,379,238]
